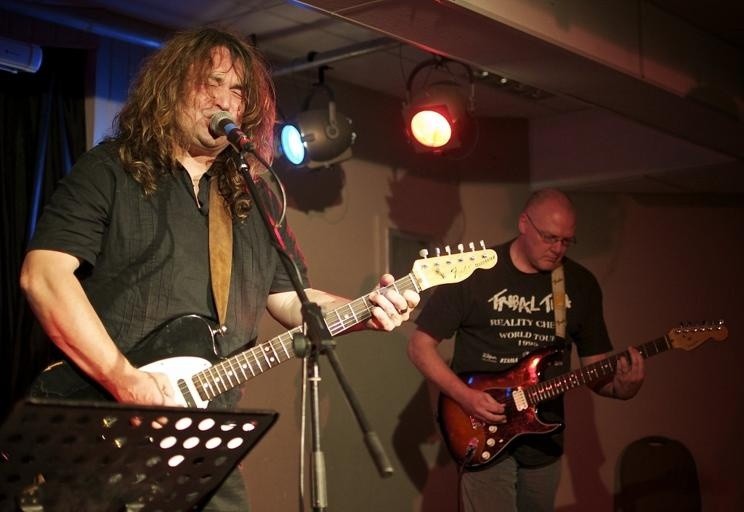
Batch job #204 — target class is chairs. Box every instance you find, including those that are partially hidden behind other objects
[619,435,701,512]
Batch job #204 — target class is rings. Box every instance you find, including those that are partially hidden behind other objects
[398,304,408,314]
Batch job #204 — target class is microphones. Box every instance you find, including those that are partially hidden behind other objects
[209,111,255,152]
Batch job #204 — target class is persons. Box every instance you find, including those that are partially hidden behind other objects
[406,190,646,512]
[16,28,422,512]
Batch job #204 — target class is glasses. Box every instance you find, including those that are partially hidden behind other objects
[526,214,577,247]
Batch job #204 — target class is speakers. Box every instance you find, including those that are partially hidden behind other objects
[0,37,44,75]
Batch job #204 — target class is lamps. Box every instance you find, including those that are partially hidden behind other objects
[279,63,363,174]
[398,56,474,155]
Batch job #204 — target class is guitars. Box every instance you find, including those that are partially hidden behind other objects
[437,318,728,471]
[31,240,499,479]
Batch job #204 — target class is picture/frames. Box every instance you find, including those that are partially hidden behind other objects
[374,212,440,322]
